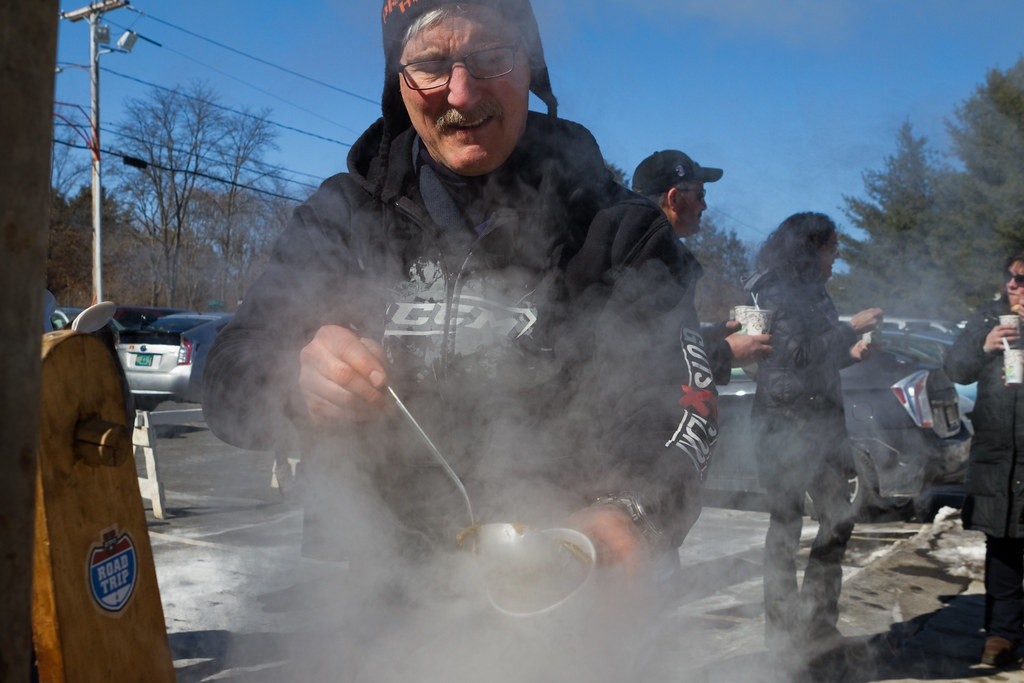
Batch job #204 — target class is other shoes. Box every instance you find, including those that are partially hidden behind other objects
[980,636,1017,666]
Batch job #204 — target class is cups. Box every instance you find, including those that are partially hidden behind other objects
[1003,349,1024,384]
[734,305,756,333]
[744,309,773,336]
[998,314,1020,335]
[486,527,626,661]
[862,318,883,346]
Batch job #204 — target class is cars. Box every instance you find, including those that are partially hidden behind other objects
[116,314,227,410]
[708,313,981,527]
[42,296,183,348]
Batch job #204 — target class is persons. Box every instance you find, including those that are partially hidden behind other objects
[943,251,1024,665]
[187,0,720,683]
[751,211,883,666]
[635,151,770,385]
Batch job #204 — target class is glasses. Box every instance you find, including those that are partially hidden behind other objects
[393,39,522,90]
[1002,271,1024,283]
[675,188,708,201]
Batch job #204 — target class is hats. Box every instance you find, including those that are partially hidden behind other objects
[632,149,724,195]
[371,0,558,202]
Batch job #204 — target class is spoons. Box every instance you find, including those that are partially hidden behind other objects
[71,301,117,333]
